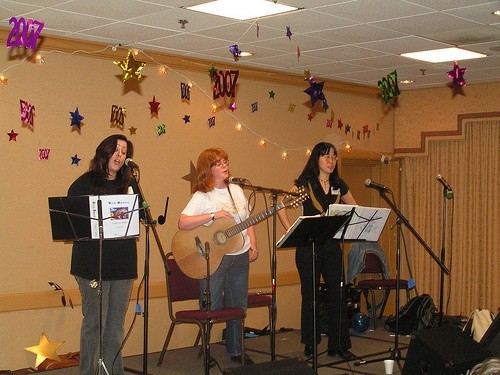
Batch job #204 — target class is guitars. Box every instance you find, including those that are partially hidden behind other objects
[171,186,311,279]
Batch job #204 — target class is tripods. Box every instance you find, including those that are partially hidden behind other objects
[305,191,450,366]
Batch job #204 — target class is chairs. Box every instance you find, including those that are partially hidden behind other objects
[216,290,274,353]
[156,252,245,372]
[351,240,413,331]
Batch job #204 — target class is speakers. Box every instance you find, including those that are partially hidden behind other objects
[401,322,488,375]
[222,357,318,375]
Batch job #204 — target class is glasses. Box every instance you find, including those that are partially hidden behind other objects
[320,154,338,161]
[211,160,230,169]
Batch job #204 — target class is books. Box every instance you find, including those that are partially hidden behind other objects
[326,204,391,241]
[276,215,322,247]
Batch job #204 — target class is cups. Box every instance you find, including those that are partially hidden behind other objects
[384,360,395,374]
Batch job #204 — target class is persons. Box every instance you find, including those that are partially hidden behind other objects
[178,148,259,365]
[68,134,145,375]
[276,142,358,359]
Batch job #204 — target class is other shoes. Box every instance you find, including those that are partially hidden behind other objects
[230,355,255,364]
[304,344,317,357]
[328,349,358,360]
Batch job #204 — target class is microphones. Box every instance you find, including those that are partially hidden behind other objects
[229,176,247,183]
[205,241,210,256]
[125,158,139,170]
[364,179,389,191]
[436,174,452,191]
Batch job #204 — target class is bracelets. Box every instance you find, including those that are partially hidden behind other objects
[211,213,214,220]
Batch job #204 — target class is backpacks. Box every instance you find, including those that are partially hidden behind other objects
[384,293,436,335]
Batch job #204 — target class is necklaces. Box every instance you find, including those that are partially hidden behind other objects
[320,179,329,185]
